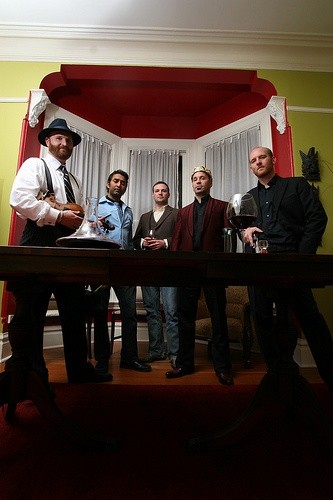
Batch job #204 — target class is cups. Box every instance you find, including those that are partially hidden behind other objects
[146,229,157,240]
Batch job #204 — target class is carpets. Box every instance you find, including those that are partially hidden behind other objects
[0,383,333,500]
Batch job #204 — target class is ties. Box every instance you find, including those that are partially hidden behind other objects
[59,166,76,205]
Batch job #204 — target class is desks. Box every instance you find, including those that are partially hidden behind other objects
[0,246,333,288]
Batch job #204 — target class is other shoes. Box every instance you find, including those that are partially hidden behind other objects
[70,371,113,381]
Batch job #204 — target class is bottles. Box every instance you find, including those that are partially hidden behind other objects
[55,197,123,250]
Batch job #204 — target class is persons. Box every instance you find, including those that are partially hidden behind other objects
[132,180,184,371]
[9,117,113,387]
[165,166,237,386]
[235,146,332,385]
[91,170,152,375]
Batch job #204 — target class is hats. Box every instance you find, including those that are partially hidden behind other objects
[38,119,81,147]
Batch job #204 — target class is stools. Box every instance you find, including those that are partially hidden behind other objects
[111,310,148,355]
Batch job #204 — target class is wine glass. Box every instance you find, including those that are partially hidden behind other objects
[227,194,256,253]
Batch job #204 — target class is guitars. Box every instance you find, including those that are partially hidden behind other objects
[37,188,85,229]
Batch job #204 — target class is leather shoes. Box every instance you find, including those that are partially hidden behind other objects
[96,359,109,371]
[122,360,153,372]
[215,369,233,384]
[166,367,191,378]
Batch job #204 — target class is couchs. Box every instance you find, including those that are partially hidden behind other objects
[195,286,258,369]
